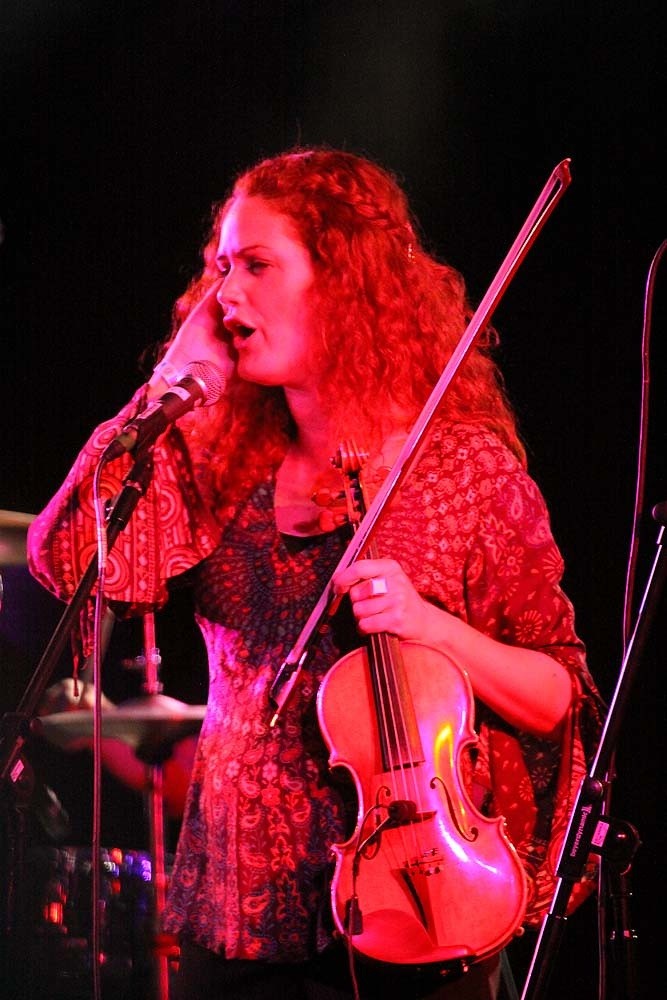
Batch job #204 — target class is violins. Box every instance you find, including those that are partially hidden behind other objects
[306,434,531,985]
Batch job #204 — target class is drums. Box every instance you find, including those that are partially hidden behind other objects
[19,843,176,975]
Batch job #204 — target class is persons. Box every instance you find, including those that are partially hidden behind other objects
[42,680,206,810]
[27,149,609,1000]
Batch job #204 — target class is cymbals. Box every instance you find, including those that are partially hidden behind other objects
[37,693,210,747]
[0,510,41,568]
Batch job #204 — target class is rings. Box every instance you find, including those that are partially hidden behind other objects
[369,577,388,597]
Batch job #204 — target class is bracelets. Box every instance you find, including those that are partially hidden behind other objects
[152,358,180,387]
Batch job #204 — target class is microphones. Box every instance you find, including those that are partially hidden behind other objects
[105,359,226,461]
